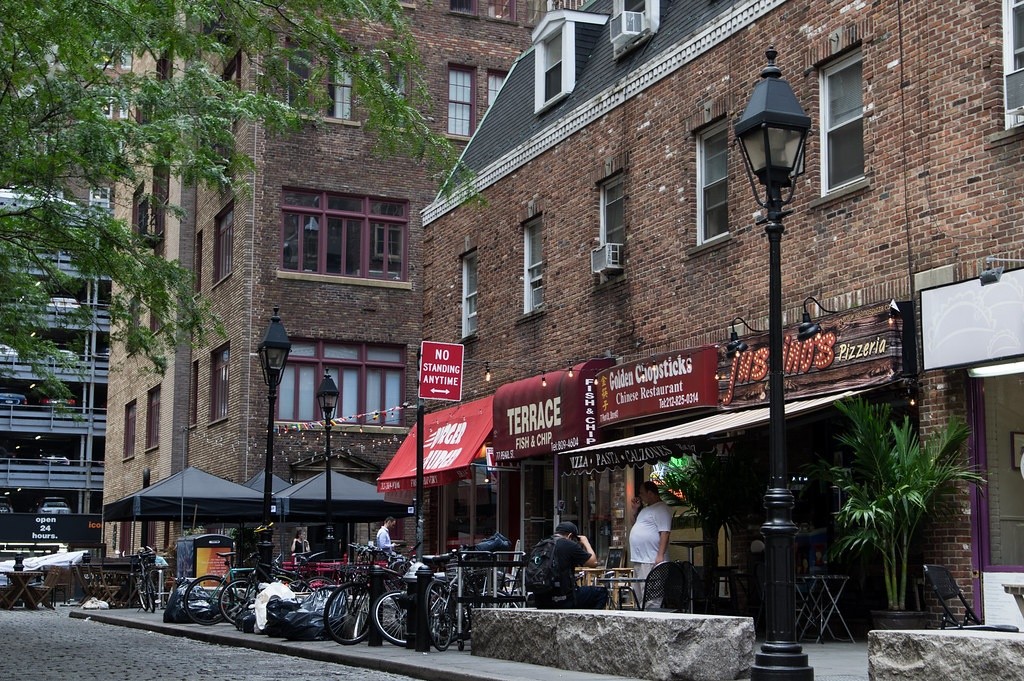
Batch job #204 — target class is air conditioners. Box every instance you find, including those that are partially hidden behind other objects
[589,242,624,276]
[609,11,646,43]
[1003,67,1024,130]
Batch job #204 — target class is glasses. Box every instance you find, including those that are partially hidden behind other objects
[391,521,395,526]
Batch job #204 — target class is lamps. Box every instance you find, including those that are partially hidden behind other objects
[980,255,1024,287]
[725,316,763,360]
[796,295,841,341]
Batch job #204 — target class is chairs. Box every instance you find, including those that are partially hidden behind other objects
[0,562,135,612]
[639,561,689,613]
[923,563,1018,631]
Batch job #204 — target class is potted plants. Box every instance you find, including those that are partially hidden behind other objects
[804,395,988,629]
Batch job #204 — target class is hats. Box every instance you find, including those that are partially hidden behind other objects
[556,522,581,542]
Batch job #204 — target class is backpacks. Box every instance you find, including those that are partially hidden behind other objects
[525,535,566,587]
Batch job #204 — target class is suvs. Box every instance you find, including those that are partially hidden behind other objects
[0,387,27,409]
[36,497,71,514]
[46,294,81,313]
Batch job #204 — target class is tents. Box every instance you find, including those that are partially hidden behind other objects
[101,466,415,607]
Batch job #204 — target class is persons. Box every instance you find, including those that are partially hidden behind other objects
[628,480,672,607]
[290,529,311,563]
[532,522,608,611]
[376,516,400,556]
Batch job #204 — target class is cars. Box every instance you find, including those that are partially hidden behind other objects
[0,496,13,513]
[42,453,69,466]
[101,348,109,360]
[54,343,79,360]
[0,446,16,462]
[39,394,77,410]
[0,343,19,355]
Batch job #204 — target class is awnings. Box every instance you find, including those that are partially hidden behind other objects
[376,396,492,495]
[492,357,616,466]
[556,378,904,477]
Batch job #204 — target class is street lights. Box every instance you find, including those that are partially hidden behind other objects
[315,366,340,564]
[732,42,814,681]
[257,305,292,572]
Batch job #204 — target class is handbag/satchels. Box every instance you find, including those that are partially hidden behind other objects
[475,533,512,553]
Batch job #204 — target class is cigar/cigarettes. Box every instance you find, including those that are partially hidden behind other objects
[636,500,641,503]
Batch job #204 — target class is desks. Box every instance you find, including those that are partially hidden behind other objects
[91,563,133,607]
[795,574,856,645]
[597,577,650,612]
[0,570,44,610]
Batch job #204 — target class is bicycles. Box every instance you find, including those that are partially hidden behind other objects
[323,542,405,646]
[218,551,338,625]
[371,542,440,647]
[423,545,524,651]
[122,546,156,613]
[184,552,258,626]
